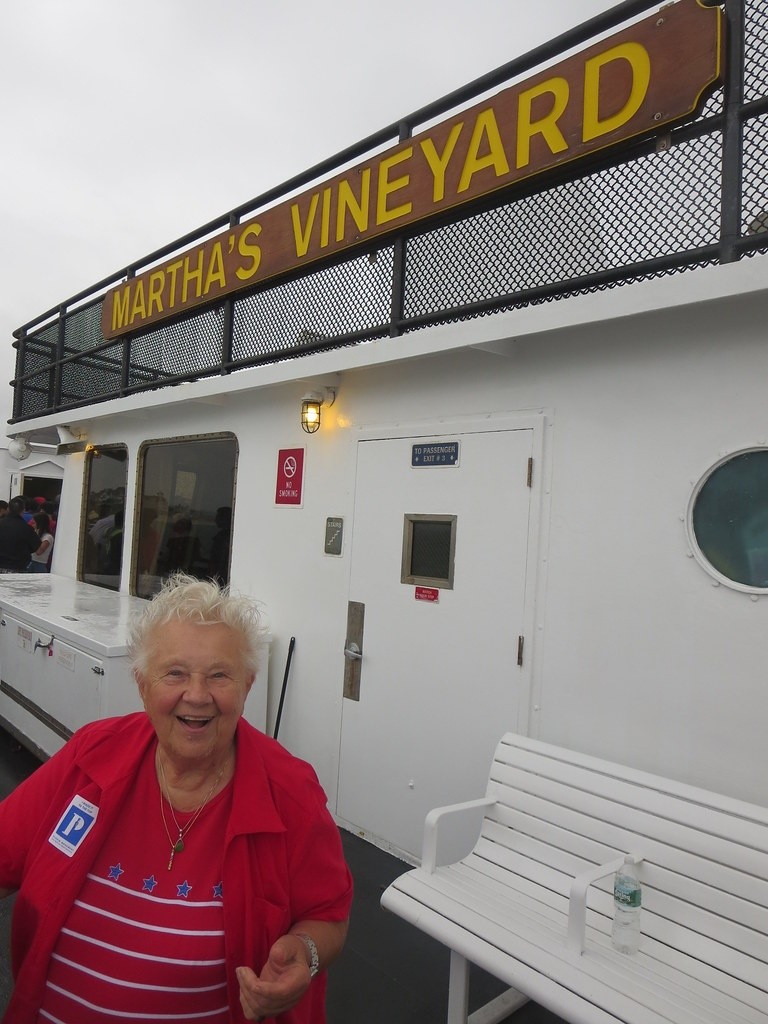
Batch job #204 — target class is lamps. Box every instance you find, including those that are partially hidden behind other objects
[10,433,33,460]
[301,387,336,432]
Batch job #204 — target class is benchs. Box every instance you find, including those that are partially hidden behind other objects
[378,728,768,1024]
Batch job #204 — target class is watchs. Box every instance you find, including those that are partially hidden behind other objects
[295,933,319,978]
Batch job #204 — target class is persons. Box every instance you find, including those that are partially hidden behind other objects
[1,574,354,1024]
[0,494,62,572]
[84,503,232,586]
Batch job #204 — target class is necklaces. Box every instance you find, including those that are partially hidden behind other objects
[158,751,225,870]
